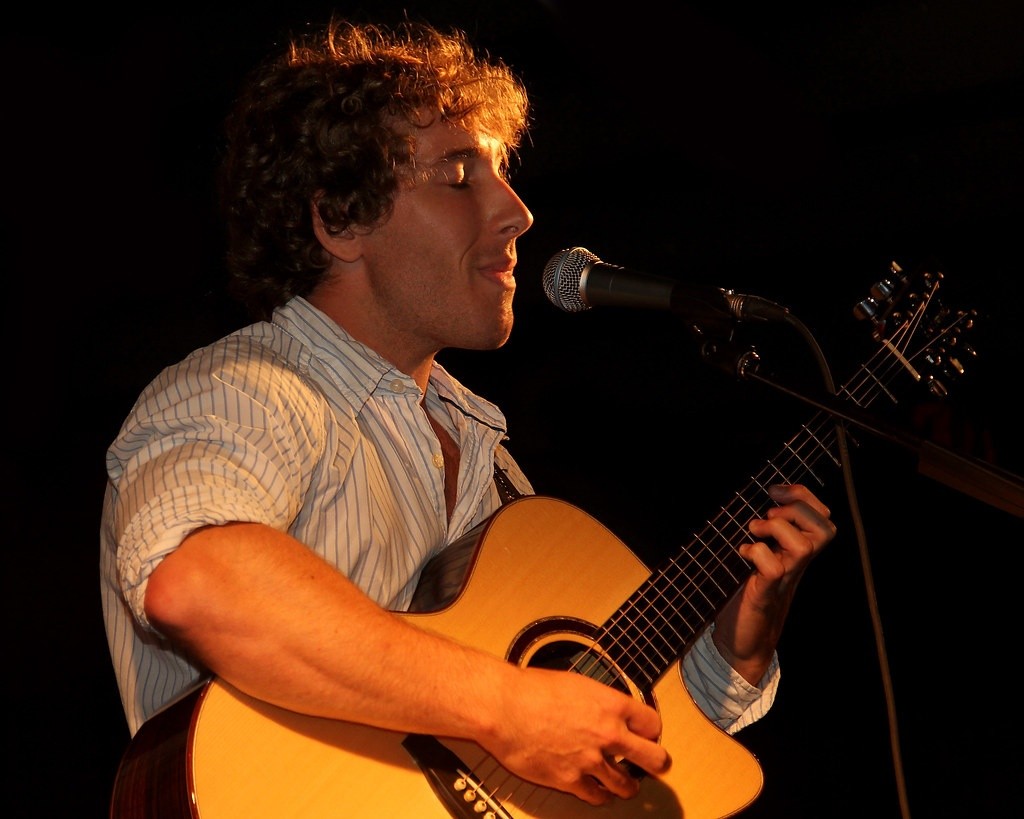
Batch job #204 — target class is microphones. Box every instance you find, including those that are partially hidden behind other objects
[543,247,789,323]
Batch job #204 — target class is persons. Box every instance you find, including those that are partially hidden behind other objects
[98,27,837,818]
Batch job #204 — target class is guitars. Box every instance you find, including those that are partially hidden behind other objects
[106,258,993,818]
